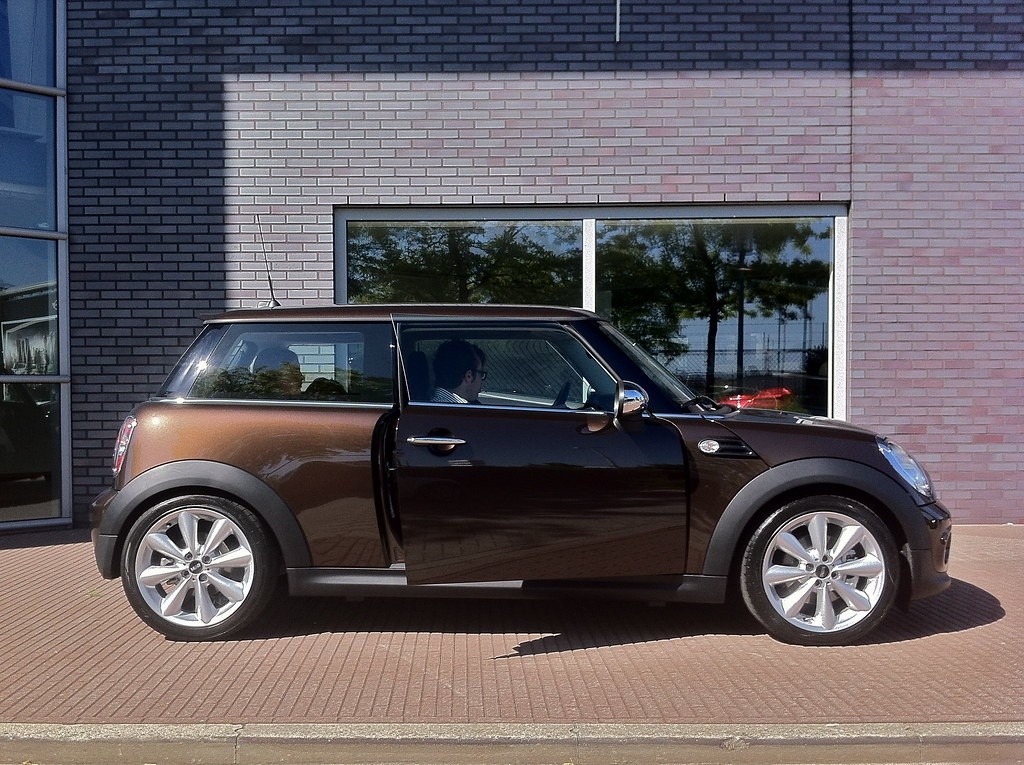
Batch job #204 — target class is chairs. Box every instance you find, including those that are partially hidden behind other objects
[406,350,430,403]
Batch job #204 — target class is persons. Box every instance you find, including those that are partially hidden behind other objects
[253,347,349,401]
[429,338,488,404]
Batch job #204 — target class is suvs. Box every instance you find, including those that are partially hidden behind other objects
[92,302,953,652]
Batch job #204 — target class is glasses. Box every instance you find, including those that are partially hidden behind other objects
[474,369,487,380]
